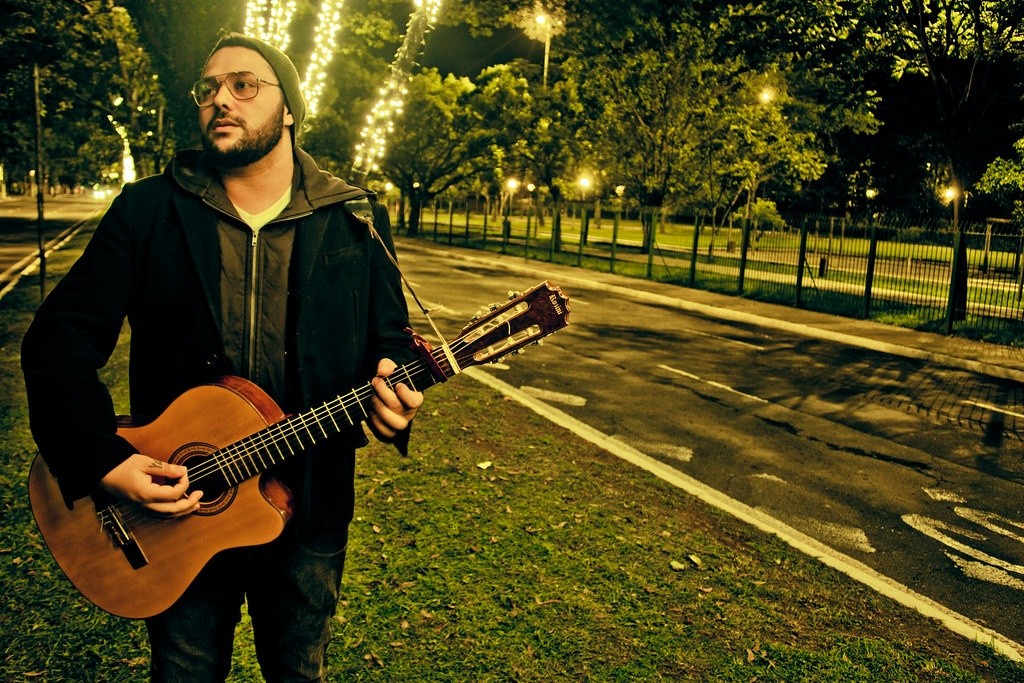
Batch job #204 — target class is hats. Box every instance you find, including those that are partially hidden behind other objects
[200,35,306,148]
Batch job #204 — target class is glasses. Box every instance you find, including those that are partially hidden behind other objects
[190,71,280,107]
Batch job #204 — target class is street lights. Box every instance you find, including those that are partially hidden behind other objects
[861,185,883,317]
[579,178,591,240]
[506,176,516,219]
[539,14,553,92]
[524,182,536,262]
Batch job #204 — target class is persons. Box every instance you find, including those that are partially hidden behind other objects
[22,32,423,683]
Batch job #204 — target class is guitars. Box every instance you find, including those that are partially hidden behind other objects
[28,279,573,619]
